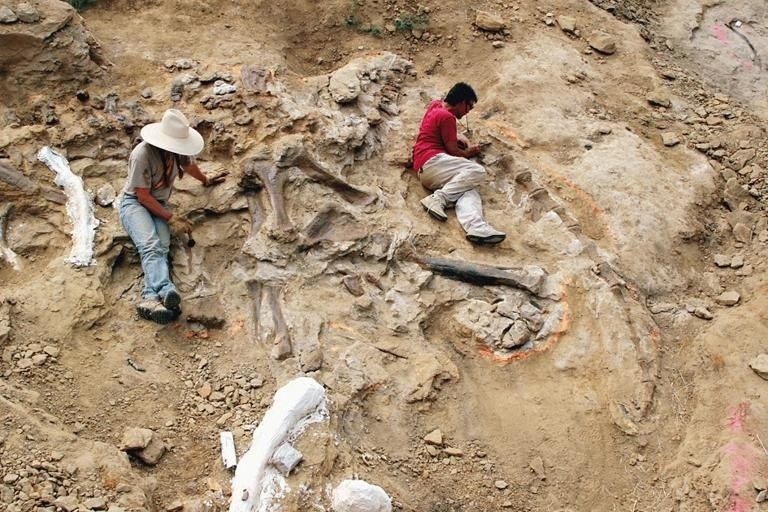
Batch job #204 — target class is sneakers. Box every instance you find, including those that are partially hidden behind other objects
[163,289,181,319]
[419,195,447,221]
[465,225,506,244]
[136,301,173,324]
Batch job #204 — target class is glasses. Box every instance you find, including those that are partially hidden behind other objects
[465,101,473,110]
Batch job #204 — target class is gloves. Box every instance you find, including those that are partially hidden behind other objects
[166,214,193,236]
[205,166,229,187]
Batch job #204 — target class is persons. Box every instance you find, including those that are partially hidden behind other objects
[410,81,507,246]
[116,109,232,326]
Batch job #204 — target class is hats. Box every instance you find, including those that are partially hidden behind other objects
[140,109,204,156]
